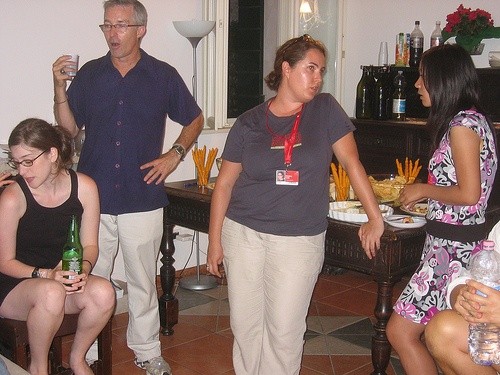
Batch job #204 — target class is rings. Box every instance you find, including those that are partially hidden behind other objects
[60,68,65,74]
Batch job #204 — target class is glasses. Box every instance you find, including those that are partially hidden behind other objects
[7,149,50,169]
[99,24,143,32]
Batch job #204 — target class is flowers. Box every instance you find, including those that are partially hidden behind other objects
[441,4,500,53]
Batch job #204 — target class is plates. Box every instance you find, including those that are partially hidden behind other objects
[399,202,428,217]
[385,214,427,229]
[327,201,394,223]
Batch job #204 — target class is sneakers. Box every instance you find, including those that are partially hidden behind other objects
[133,356,172,375]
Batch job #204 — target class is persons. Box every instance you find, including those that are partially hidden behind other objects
[386,44,498,375]
[0,118,116,375]
[424,220,500,375]
[52,0,204,375]
[277,172,284,181]
[206,34,385,375]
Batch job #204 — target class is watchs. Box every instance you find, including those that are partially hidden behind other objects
[172,144,185,161]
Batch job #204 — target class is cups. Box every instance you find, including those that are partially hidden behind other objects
[194,167,211,189]
[335,186,350,200]
[378,41,388,66]
[65,54,79,76]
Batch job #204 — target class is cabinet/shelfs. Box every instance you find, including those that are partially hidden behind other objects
[348,67,500,204]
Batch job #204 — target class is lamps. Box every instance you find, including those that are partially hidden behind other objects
[172,20,218,290]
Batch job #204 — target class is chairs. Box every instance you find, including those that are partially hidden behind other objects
[0,170,111,375]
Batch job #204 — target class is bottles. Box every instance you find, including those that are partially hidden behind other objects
[469,240,500,367]
[394,32,411,67]
[408,21,425,68]
[62,215,82,291]
[356,65,408,119]
[431,21,444,48]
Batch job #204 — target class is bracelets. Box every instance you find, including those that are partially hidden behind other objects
[32,267,39,278]
[83,260,92,268]
[54,96,67,104]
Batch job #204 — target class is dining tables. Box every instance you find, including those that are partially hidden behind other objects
[159,171,500,375]
